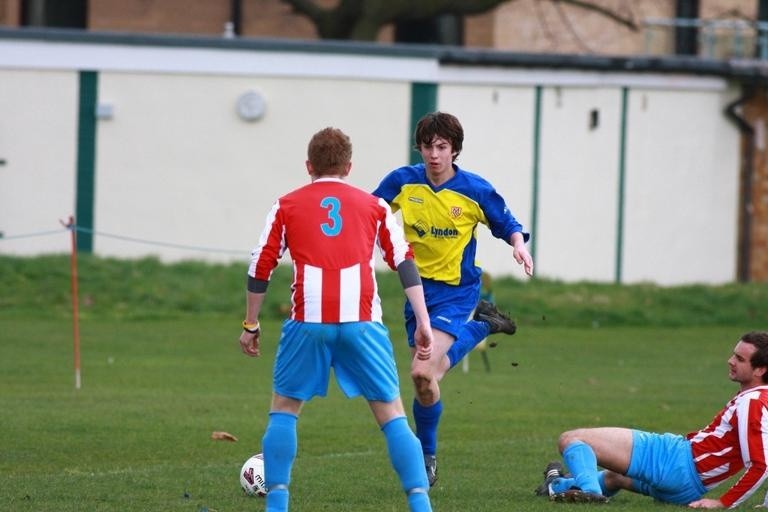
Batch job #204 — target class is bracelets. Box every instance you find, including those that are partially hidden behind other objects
[242,320,262,333]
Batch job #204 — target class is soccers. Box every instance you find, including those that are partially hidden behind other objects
[241,453,268,497]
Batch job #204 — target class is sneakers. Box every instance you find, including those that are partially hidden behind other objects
[424,455,437,486]
[474,300,515,334]
[535,463,607,503]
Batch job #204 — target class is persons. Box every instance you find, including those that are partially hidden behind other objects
[239,128,434,511]
[372,111,534,487]
[535,331,768,510]
